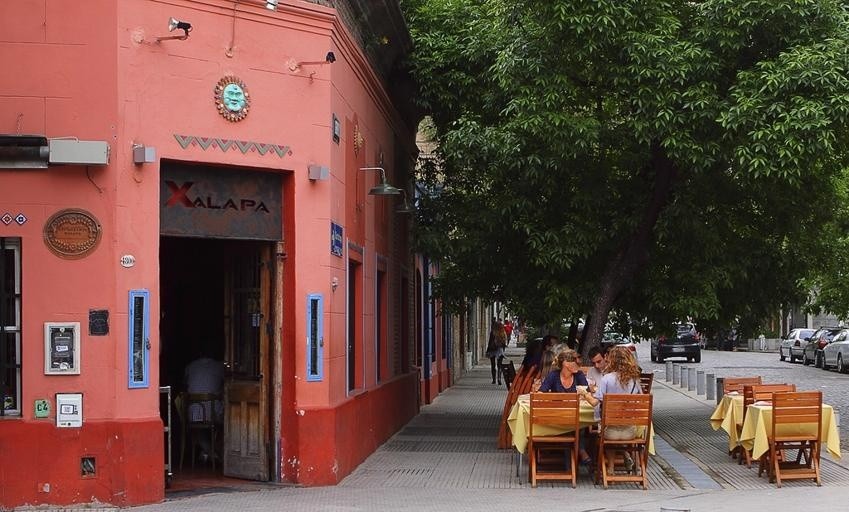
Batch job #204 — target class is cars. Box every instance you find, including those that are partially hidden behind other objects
[803,326,844,367]
[599,330,637,360]
[650,321,702,364]
[821,328,849,373]
[779,328,818,362]
[562,316,586,337]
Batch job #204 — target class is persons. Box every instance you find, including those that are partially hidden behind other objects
[503,322,513,344]
[485,323,507,385]
[183,345,224,465]
[522,335,645,470]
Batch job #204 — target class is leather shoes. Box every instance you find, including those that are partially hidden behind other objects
[579,456,593,465]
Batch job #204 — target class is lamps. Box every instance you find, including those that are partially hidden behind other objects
[359,167,398,199]
[156,16,194,43]
[299,52,336,70]
[393,183,417,216]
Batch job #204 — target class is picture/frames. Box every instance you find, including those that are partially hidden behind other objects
[55,392,83,428]
[44,322,81,376]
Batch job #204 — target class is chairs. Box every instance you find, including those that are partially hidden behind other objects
[722,375,822,488]
[527,364,654,491]
[174,391,224,474]
[500,361,516,392]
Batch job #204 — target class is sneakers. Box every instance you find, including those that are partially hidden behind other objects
[624,457,634,470]
